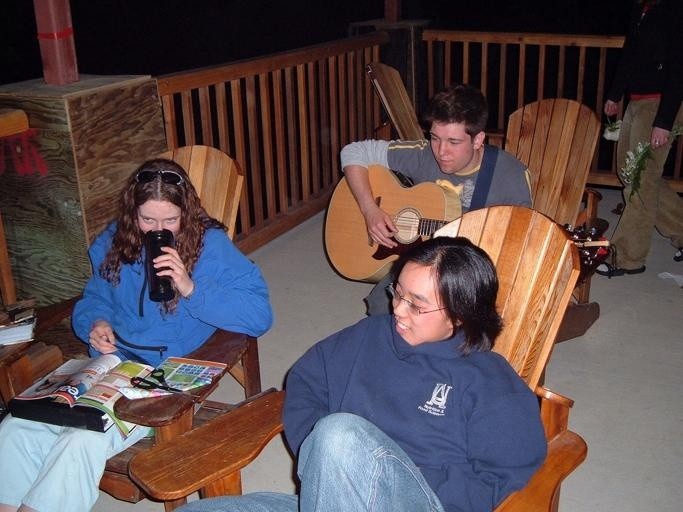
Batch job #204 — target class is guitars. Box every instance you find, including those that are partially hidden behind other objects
[326,165,612,284]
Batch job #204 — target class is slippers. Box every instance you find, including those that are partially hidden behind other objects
[673,248,683,261]
[596,261,646,276]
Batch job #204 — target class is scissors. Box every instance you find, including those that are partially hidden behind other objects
[131,369,200,404]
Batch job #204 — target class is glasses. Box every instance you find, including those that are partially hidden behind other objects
[387,282,447,315]
[135,170,187,187]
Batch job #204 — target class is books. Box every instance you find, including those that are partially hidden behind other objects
[7,354,166,439]
[1,298,38,346]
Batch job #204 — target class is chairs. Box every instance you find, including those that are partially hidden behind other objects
[505,98,610,344]
[127,205,588,512]
[365,61,425,141]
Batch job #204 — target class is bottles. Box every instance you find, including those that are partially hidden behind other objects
[144,228,176,302]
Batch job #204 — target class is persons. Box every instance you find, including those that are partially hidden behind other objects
[339,84,535,318]
[173,235,549,512]
[594,1,683,277]
